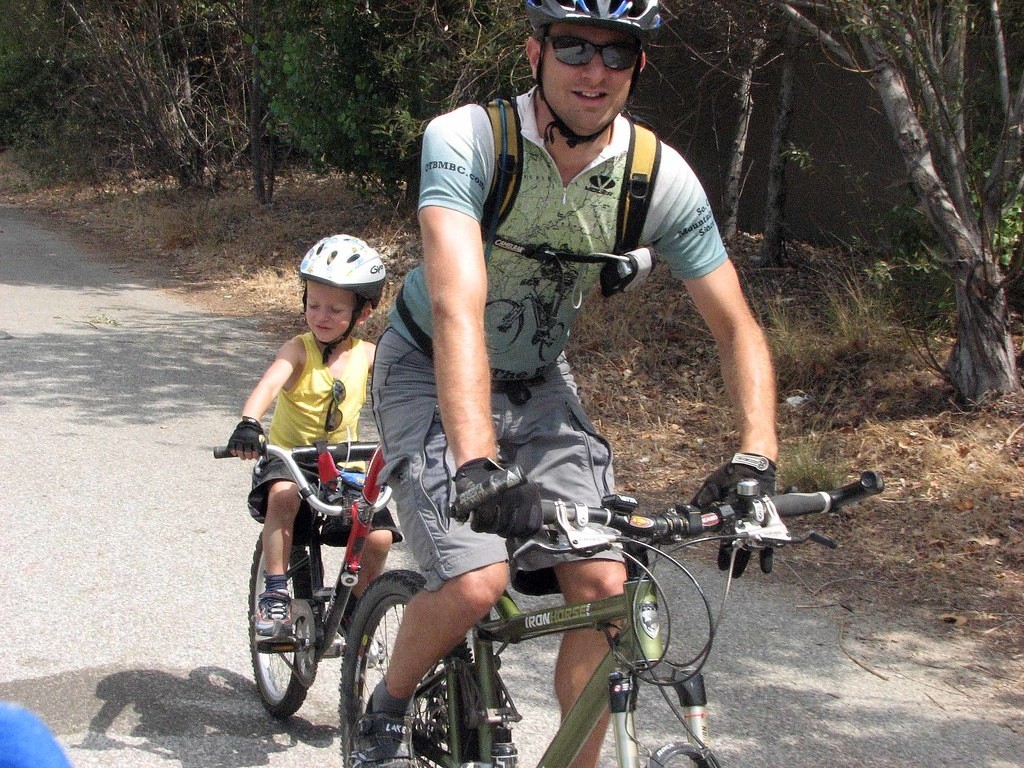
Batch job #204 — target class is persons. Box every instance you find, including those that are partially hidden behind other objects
[345,0,777,768]
[220,232,404,669]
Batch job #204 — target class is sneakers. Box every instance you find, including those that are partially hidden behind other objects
[338,607,383,670]
[356,696,415,768]
[251,589,302,646]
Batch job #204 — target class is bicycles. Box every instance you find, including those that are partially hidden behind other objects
[214,434,886,768]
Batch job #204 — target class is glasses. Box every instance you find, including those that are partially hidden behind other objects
[541,36,642,71]
[325,378,347,435]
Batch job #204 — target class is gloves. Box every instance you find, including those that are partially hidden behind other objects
[454,458,548,539]
[689,452,781,580]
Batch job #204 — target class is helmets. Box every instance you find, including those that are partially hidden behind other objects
[298,233,387,309]
[526,2,664,46]
